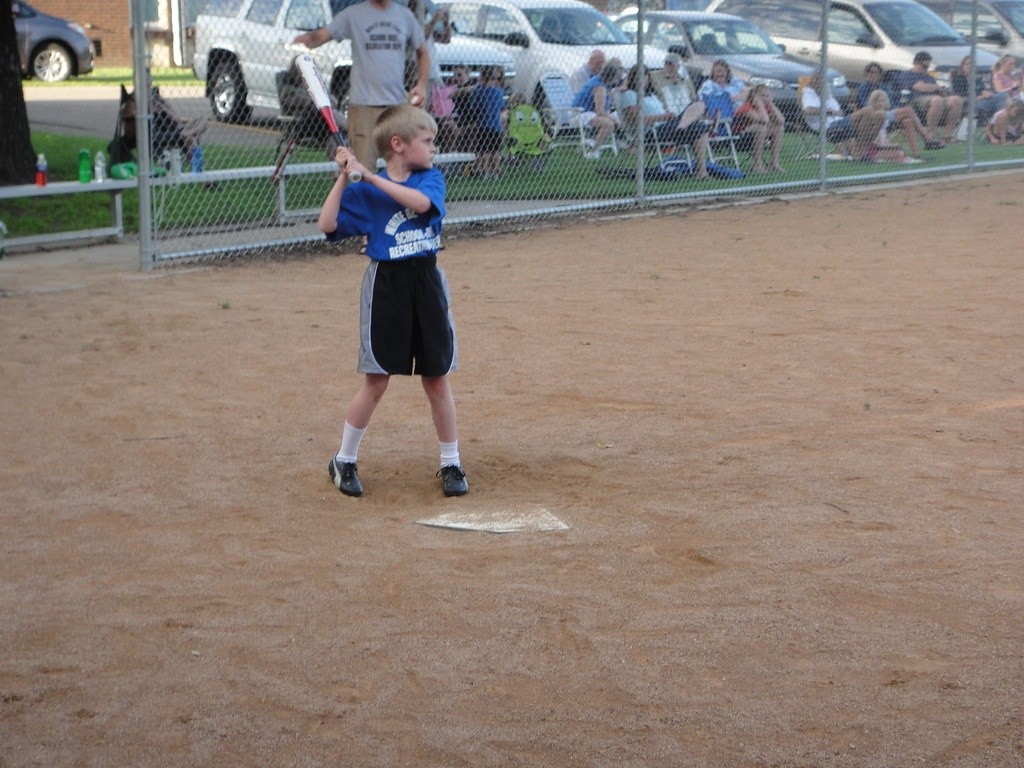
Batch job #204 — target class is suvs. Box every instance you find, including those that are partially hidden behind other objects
[191,0,518,125]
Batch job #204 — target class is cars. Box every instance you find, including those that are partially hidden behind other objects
[434,1,691,133]
[597,11,852,122]
[13,0,95,82]
[913,0,1024,61]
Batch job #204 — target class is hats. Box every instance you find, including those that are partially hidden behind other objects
[663,53,682,65]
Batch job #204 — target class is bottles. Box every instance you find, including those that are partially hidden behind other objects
[93,150,106,182]
[78,149,92,183]
[35,153,48,186]
[191,146,202,174]
[170,147,181,176]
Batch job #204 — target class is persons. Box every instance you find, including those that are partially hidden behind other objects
[802,51,1024,162]
[318,104,469,496]
[570,49,786,179]
[291,0,428,253]
[407,0,558,181]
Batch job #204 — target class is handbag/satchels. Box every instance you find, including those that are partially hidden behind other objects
[953,117,977,142]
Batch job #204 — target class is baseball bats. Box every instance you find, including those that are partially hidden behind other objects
[293,51,364,183]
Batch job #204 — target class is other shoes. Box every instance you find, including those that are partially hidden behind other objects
[694,176,720,183]
[753,162,786,175]
[585,139,600,158]
[924,140,943,149]
[481,170,499,181]
[677,102,706,129]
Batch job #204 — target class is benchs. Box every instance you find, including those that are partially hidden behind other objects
[0,152,475,250]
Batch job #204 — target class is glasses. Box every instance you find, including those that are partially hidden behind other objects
[918,62,929,69]
[497,76,503,82]
[457,73,461,77]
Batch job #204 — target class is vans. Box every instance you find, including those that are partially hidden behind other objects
[695,0,1000,102]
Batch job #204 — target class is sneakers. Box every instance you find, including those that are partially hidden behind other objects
[327,452,362,497]
[436,464,468,497]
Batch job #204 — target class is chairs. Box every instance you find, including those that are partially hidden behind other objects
[541,72,740,174]
[273,70,331,166]
[795,77,834,162]
[107,85,170,174]
